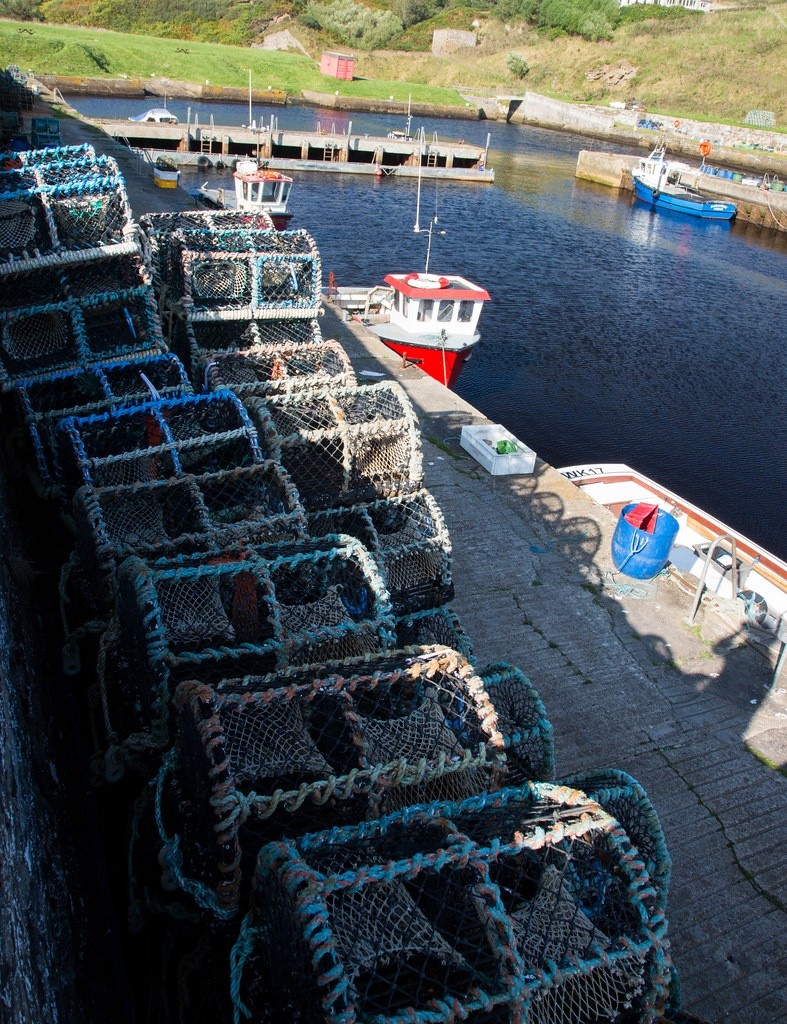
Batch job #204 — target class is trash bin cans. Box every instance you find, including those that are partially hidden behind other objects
[612,501,679,580]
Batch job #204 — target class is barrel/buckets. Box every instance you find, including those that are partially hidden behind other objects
[611,504,679,580]
[701,166,784,192]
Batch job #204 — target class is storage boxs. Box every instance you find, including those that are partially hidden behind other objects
[153,167,178,189]
[624,502,659,535]
[692,541,751,589]
[460,424,537,476]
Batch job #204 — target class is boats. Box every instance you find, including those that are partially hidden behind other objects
[556,463,787,656]
[196,120,295,230]
[317,273,491,392]
[629,142,737,221]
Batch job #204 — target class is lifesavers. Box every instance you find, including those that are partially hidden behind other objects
[404,272,450,288]
[674,120,680,127]
[699,141,711,156]
